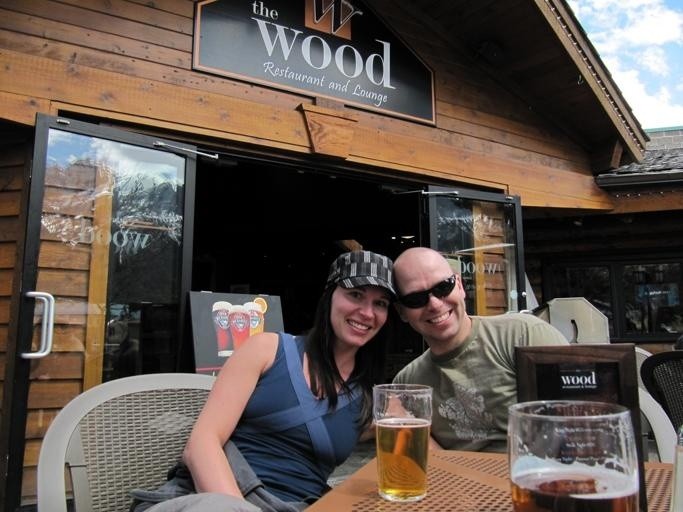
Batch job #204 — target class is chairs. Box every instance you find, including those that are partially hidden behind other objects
[34,372,216,511]
[635,347,682,436]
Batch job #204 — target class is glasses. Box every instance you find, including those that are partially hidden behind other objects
[398,273,457,309]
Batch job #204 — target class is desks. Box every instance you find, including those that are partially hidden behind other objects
[299,449,675,511]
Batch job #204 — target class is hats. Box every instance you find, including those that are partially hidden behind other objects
[324,250,397,296]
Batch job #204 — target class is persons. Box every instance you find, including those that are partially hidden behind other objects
[393,245,573,454]
[131,250,396,512]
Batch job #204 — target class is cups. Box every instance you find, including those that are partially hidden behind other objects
[210,301,234,357]
[373,381,433,504]
[242,301,264,336]
[228,306,249,351]
[503,397,641,510]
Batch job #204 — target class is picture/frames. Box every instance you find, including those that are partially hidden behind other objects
[513,341,651,511]
